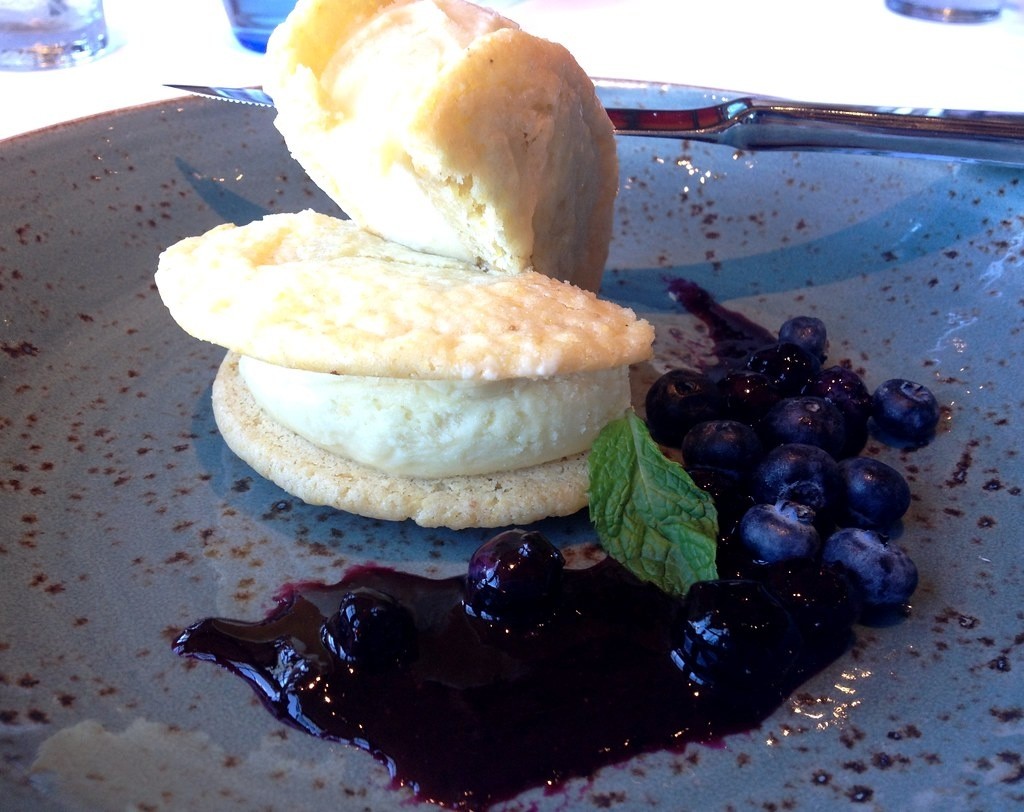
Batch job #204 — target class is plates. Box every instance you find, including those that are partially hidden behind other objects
[0,78,1024,812]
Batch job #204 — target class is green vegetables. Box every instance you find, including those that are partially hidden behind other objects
[584,406,721,595]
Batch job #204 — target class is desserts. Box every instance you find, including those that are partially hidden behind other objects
[154,208,656,532]
[260,0,620,296]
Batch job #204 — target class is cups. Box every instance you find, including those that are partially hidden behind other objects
[223,1,297,53]
[0,0,108,71]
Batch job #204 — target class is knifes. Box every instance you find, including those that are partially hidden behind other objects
[163,82,1023,140]
